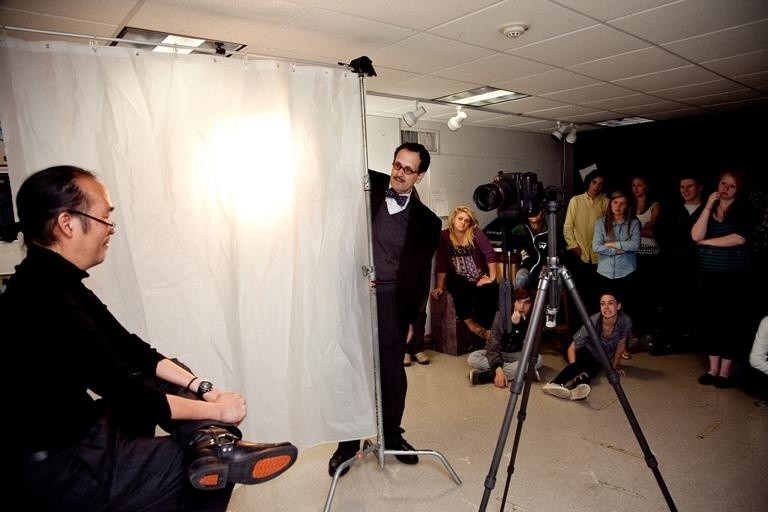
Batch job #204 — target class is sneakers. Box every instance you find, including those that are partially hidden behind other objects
[468,368,496,385]
[542,382,570,399]
[567,384,591,401]
[524,370,542,383]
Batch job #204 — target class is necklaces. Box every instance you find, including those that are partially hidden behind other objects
[719,204,726,217]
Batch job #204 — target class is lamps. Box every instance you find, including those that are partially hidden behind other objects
[400,98,579,146]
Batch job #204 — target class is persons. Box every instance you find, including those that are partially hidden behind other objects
[327,143,443,478]
[466,288,546,388]
[749,315,768,374]
[562,169,610,264]
[512,210,549,291]
[631,176,660,346]
[541,291,632,403]
[403,290,432,366]
[690,171,754,389]
[430,205,499,340]
[650,176,705,356]
[0,166,299,511]
[593,190,642,360]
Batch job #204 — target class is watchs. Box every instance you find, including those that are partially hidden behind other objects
[197,380,214,400]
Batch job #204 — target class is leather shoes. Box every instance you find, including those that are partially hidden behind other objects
[622,351,631,360]
[383,434,419,465]
[328,442,360,478]
[411,351,430,365]
[698,372,734,389]
[403,353,412,366]
[185,426,298,492]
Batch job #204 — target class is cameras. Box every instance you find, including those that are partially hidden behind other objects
[473,171,542,218]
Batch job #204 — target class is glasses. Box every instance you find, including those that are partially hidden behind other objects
[66,209,117,234]
[392,161,419,175]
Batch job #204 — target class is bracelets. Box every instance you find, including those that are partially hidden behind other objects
[489,277,493,283]
[185,376,199,391]
[704,206,711,210]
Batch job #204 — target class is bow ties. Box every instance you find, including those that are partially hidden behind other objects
[384,187,409,207]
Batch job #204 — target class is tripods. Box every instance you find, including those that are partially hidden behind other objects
[479,201,678,512]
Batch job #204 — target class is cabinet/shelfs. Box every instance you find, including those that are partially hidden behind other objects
[429,288,485,357]
